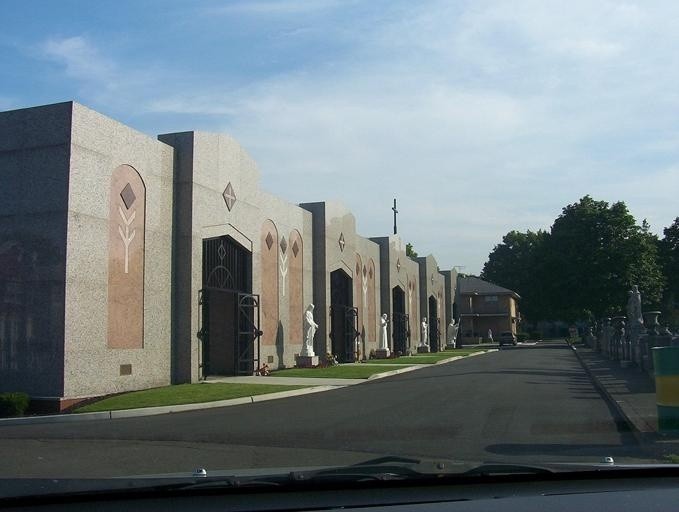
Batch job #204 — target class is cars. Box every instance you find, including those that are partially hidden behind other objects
[499,330,518,346]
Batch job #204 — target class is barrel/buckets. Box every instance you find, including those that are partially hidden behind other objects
[650,344,679,438]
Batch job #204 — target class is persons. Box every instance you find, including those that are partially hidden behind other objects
[421,317,429,346]
[447,319,459,344]
[301,303,319,356]
[378,313,389,349]
[487,328,493,344]
